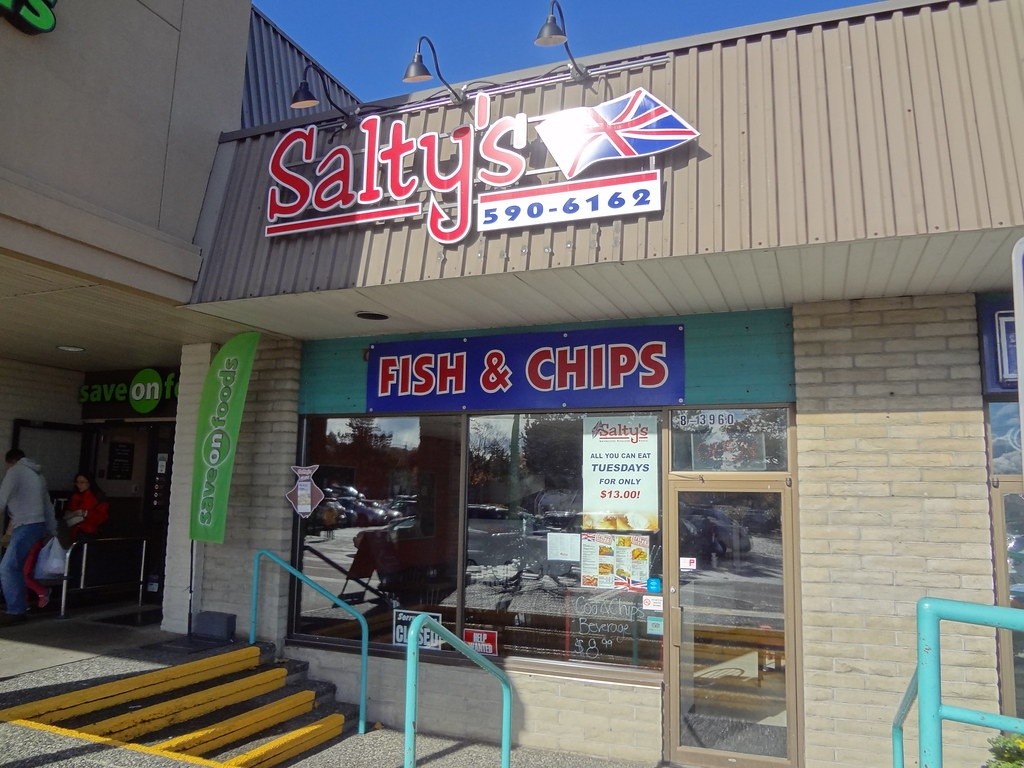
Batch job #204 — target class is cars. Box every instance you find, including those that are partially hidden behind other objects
[310,484,752,610]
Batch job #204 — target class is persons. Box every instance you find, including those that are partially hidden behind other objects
[3,517,52,611]
[65,471,111,594]
[0,448,59,628]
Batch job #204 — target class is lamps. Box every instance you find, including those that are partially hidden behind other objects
[402,36,462,104]
[534,0,585,77]
[290,64,352,120]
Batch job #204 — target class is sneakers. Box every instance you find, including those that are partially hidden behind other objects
[1,613,26,627]
[38,586,52,607]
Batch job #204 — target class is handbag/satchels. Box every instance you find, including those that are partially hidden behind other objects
[34,535,65,580]
[61,509,85,527]
